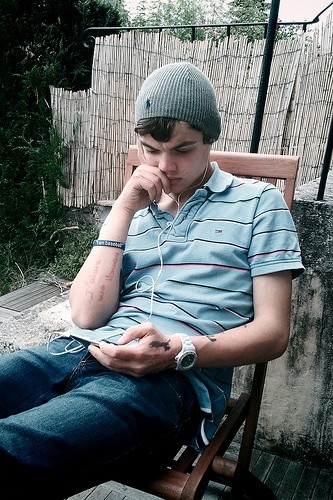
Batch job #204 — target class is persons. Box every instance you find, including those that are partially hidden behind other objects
[0,62,306,500]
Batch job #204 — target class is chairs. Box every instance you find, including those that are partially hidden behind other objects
[105,145,300,500]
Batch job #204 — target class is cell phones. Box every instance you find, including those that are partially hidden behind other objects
[102,331,143,347]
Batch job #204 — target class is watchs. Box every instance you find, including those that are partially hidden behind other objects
[173,332,199,372]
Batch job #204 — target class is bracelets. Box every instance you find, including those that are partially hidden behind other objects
[89,239,126,251]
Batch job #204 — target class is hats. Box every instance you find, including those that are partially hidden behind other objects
[135,61,222,142]
